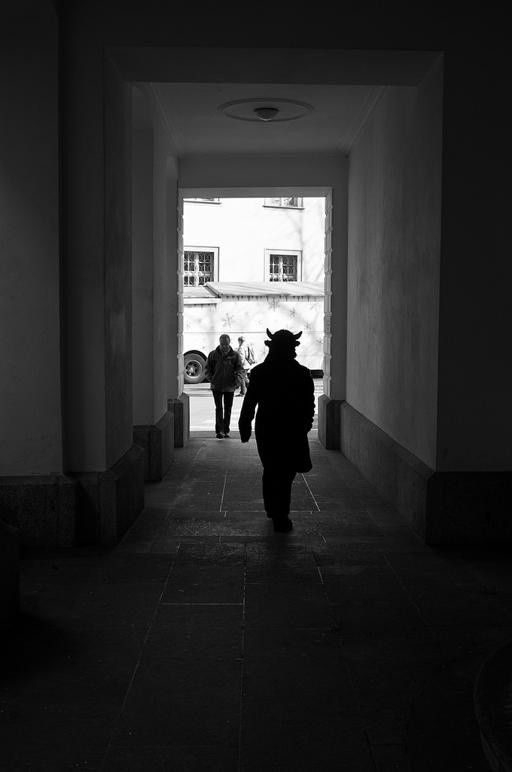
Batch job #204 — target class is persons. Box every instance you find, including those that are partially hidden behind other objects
[203,334,243,438]
[237,326,315,532]
[233,334,251,397]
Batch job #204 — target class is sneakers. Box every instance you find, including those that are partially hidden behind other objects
[216,432,229,438]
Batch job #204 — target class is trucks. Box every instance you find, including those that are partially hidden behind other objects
[182,277,325,384]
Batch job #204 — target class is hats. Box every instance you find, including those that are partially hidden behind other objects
[264,329,302,346]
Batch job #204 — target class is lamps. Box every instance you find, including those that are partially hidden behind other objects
[252,108,280,122]
